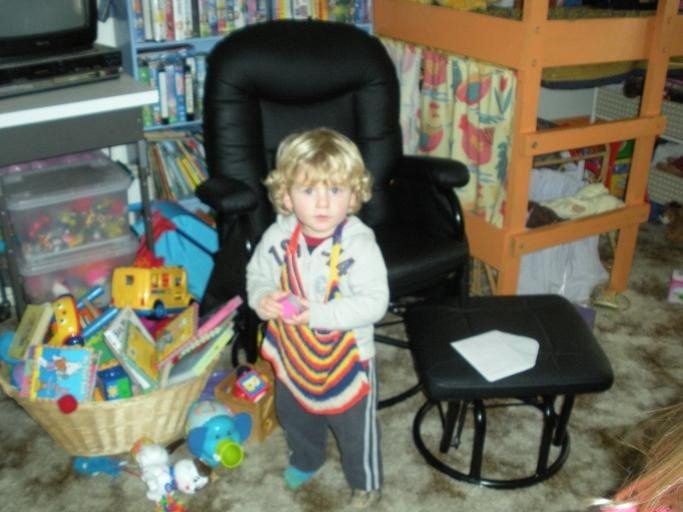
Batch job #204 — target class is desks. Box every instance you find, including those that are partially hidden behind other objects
[0,75,160,321]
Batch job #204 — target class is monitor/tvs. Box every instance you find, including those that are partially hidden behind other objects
[0,0,99,57]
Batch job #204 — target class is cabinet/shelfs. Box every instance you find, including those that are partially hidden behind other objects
[375,0,682,294]
[115,0,373,210]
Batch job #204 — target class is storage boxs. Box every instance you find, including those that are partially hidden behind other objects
[0,149,143,304]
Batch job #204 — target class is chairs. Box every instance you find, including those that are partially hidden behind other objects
[193,18,472,409]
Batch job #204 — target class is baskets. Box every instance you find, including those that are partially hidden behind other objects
[0,353,221,458]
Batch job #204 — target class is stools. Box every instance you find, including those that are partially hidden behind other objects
[403,294,615,491]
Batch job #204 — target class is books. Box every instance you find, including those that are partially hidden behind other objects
[133,0,371,43]
[138,54,211,200]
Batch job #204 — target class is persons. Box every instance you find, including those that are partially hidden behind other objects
[244,125,392,511]
[579,398,682,511]
